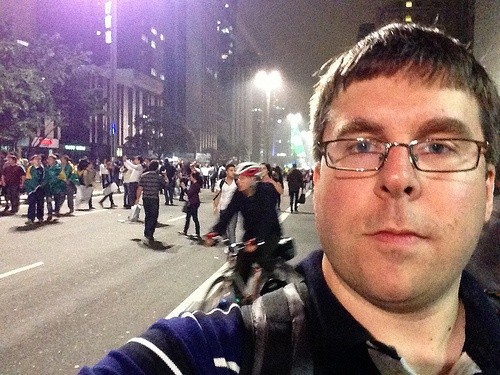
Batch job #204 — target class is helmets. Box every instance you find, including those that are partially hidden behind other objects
[232,162,264,179]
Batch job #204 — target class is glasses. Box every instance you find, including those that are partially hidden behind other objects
[316,136,491,173]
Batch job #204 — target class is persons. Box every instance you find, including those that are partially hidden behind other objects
[0,145,310,252]
[206,165,282,308]
[78,21,500,374]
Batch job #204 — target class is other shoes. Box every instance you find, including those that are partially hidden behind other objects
[277,205,300,214]
[0,178,242,271]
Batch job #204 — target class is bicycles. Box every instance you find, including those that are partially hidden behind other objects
[199,233,300,314]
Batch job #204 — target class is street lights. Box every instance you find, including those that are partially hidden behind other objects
[254,69,283,167]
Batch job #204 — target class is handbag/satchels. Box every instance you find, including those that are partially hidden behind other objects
[296,188,306,204]
